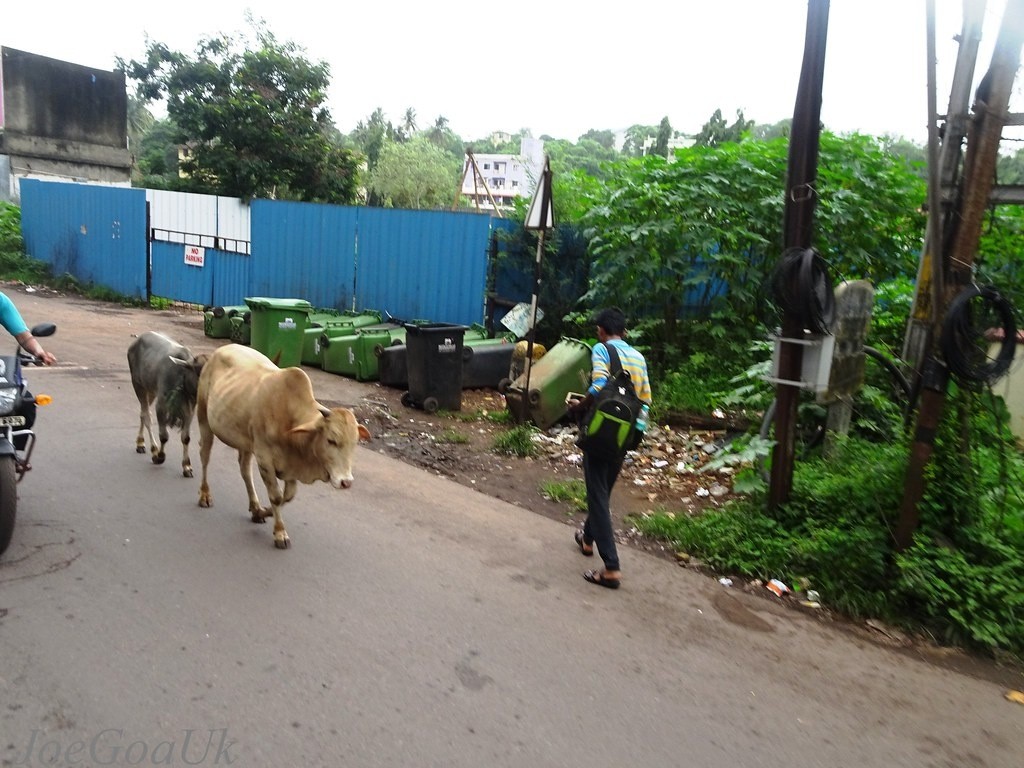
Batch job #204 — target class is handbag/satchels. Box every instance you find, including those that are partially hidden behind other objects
[576,343,650,465]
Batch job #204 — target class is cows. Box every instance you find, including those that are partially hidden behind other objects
[196,343,374,548]
[126,329,209,479]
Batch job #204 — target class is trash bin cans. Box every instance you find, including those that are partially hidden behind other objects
[400,323,469,415]
[202,302,515,395]
[242,296,314,369]
[498,336,594,430]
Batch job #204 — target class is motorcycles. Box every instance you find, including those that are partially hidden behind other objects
[0,321,59,560]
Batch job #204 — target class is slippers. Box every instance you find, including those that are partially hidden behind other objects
[575,528,594,556]
[583,569,620,589]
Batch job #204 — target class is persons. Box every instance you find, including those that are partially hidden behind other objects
[0,291,56,471]
[565,306,652,589]
